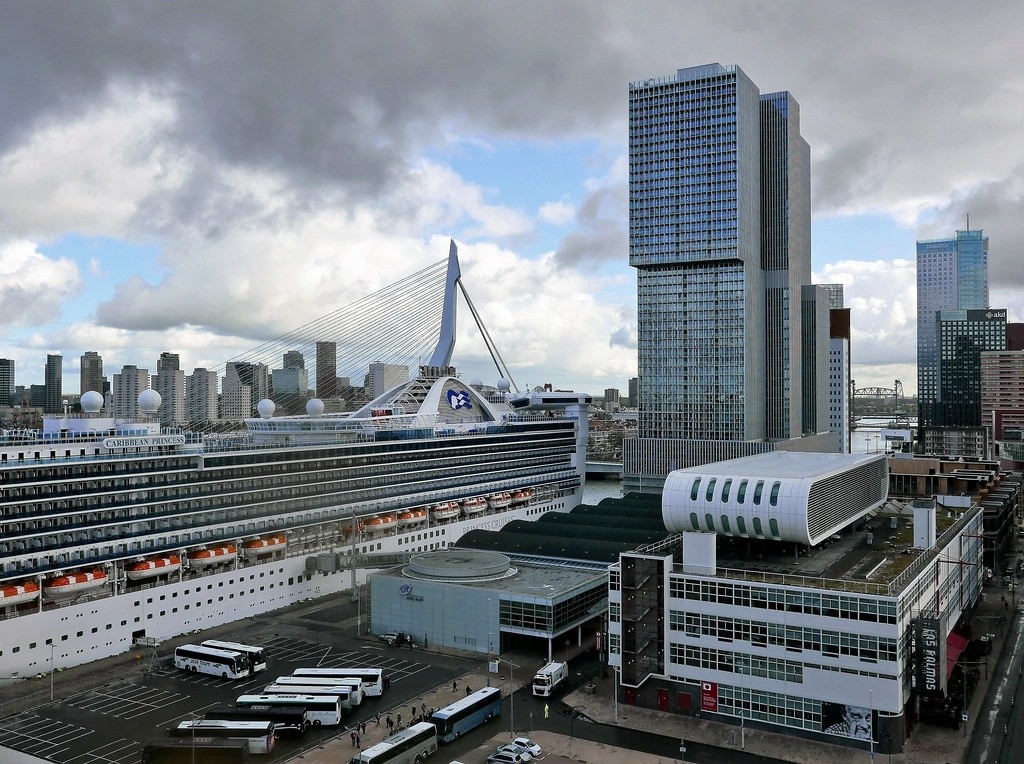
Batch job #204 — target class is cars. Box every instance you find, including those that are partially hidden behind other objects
[1001,562,1024,592]
[512,737,542,756]
[497,745,533,763]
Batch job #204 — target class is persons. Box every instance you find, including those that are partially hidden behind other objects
[387,632,411,648]
[544,704,549,718]
[350,675,473,749]
[1004,601,1009,610]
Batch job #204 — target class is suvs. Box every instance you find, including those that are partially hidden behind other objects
[486,752,521,764]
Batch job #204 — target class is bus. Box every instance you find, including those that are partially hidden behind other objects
[174,645,251,680]
[204,705,308,739]
[201,639,266,673]
[264,685,355,716]
[138,737,249,764]
[293,667,384,698]
[163,720,276,755]
[274,677,363,706]
[431,687,503,743]
[351,722,438,764]
[236,695,342,728]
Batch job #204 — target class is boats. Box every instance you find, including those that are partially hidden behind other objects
[0,580,41,608]
[126,556,181,581]
[363,489,532,532]
[44,569,108,597]
[189,544,237,566]
[242,535,290,557]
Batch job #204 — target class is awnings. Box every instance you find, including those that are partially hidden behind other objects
[944,632,969,683]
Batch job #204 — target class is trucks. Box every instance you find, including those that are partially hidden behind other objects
[532,662,569,698]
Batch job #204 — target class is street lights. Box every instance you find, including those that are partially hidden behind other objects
[612,648,618,724]
[486,632,496,686]
[869,689,874,764]
[739,667,745,751]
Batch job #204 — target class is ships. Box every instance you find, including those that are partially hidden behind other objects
[1,239,594,691]
